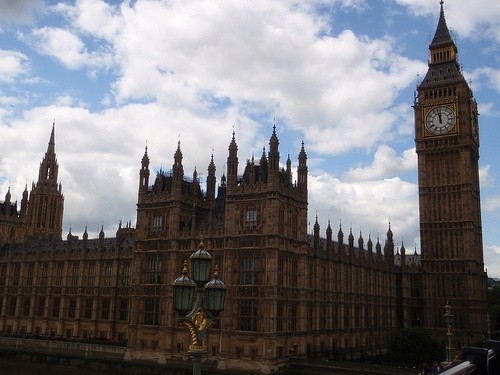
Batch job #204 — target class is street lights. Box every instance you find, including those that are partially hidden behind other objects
[443,300,452,363]
[171,234,228,375]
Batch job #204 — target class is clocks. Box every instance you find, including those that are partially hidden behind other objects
[425,105,456,135]
